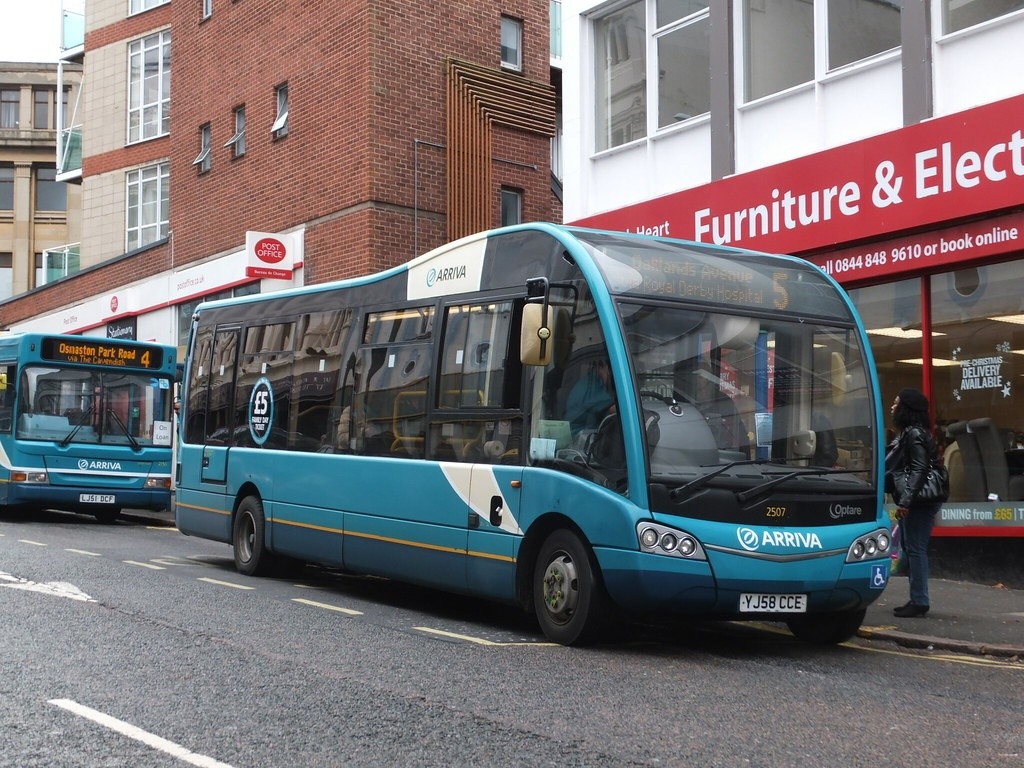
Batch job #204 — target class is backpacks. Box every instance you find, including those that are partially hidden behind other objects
[583,408,660,470]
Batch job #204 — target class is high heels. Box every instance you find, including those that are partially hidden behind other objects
[894,605,929,617]
[894,601,911,611]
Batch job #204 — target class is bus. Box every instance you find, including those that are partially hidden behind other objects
[173,220,893,649]
[0,333,185,522]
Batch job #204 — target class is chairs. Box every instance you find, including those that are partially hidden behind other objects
[548,364,581,421]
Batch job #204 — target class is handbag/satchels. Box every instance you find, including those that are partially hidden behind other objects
[891,426,949,503]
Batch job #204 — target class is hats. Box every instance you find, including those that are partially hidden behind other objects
[898,388,929,411]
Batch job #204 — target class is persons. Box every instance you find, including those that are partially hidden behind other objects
[564,358,619,434]
[880,387,941,617]
[807,410,840,470]
[338,378,391,450]
